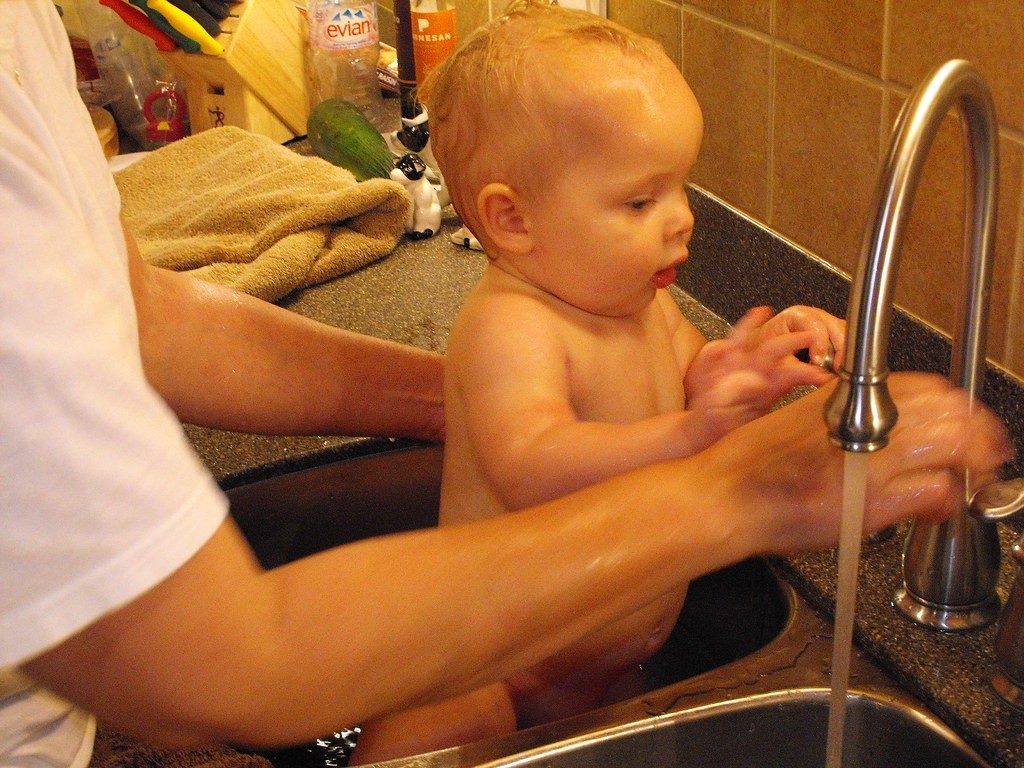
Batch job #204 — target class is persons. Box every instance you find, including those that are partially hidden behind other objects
[345,0,850,765]
[0,0,1016,768]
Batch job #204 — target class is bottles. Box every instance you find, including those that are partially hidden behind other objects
[411,1,460,103]
[307,1,385,128]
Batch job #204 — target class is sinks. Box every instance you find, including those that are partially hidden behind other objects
[489,683,996,768]
[219,436,796,725]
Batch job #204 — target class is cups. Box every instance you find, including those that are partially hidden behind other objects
[90,25,187,148]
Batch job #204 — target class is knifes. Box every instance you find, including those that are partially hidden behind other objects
[99,0,243,55]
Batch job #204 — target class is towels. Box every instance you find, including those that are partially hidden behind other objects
[112,126,414,302]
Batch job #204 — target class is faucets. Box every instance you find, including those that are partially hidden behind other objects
[818,56,1005,632]
